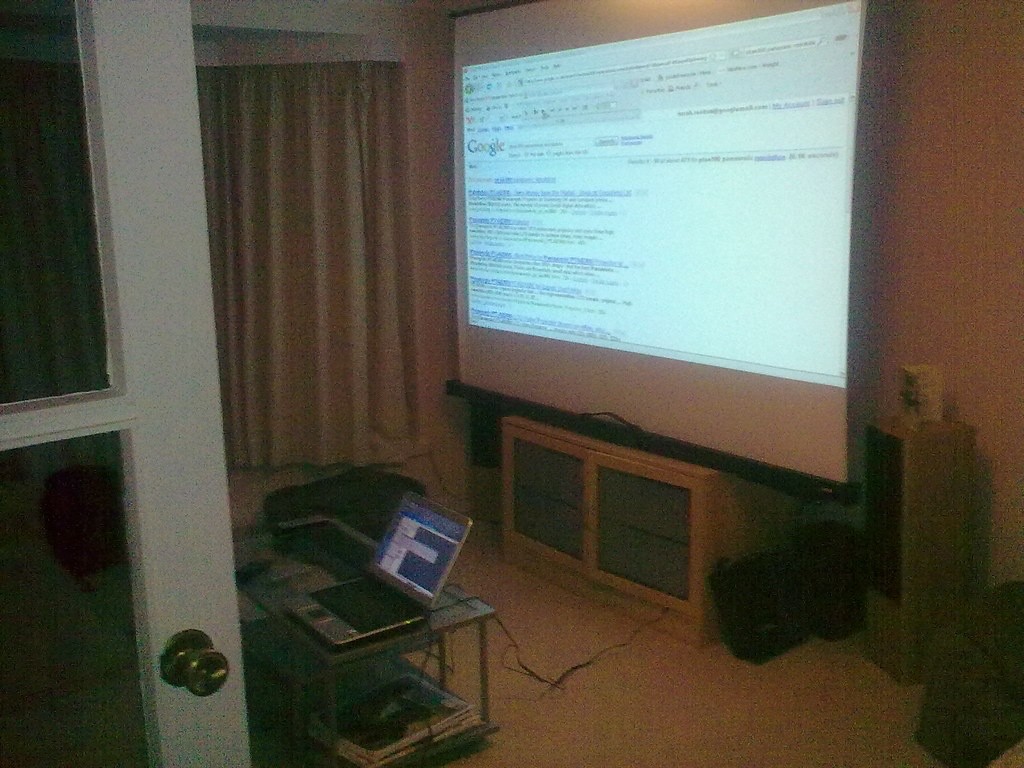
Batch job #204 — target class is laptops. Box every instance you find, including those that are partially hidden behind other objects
[281,491,473,652]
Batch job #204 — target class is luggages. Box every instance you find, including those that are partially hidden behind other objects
[913,575,1021,768]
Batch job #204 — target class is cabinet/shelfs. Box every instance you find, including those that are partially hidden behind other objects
[501,415,765,647]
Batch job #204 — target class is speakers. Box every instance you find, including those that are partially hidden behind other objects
[860,419,981,683]
[469,404,510,527]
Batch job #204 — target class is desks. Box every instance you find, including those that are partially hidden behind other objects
[235,534,496,768]
